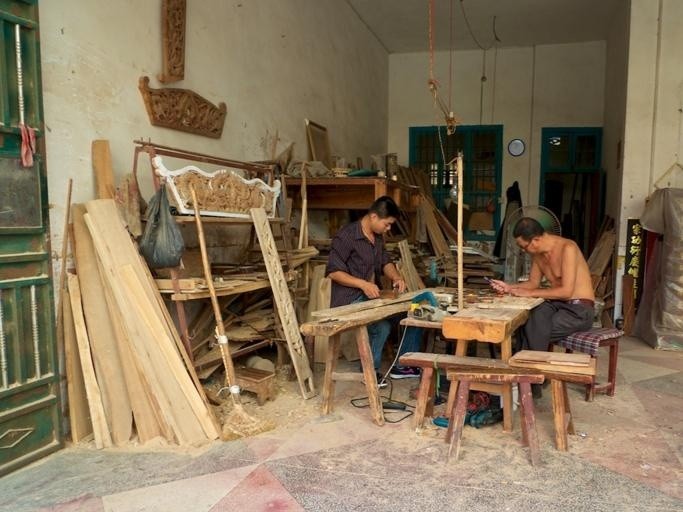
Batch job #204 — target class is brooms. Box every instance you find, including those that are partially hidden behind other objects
[187,182,277,442]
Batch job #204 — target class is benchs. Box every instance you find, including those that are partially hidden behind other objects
[299,285,457,428]
[397,347,596,469]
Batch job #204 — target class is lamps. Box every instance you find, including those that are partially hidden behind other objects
[421,2,461,135]
[444,158,463,202]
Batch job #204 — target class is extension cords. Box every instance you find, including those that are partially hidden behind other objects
[375,400,410,410]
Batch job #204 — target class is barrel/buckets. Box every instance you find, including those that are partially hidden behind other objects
[488,384,520,412]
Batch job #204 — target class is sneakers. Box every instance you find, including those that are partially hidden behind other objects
[359,366,421,388]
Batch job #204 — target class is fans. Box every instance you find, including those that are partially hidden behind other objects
[501,203,565,284]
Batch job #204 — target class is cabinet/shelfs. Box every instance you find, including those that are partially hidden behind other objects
[129,136,301,388]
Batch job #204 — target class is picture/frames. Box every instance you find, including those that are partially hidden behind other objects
[303,116,333,175]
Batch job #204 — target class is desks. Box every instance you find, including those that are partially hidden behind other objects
[257,175,422,255]
[398,279,543,432]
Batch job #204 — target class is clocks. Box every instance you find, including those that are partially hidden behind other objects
[507,138,526,158]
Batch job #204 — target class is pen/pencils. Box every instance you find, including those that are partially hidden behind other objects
[484,276,493,284]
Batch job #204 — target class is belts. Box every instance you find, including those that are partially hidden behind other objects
[566,299,594,307]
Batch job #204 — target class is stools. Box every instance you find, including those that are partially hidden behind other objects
[560,327,623,401]
[220,362,276,408]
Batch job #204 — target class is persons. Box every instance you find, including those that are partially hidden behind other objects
[324,195,422,387]
[490,216,596,398]
